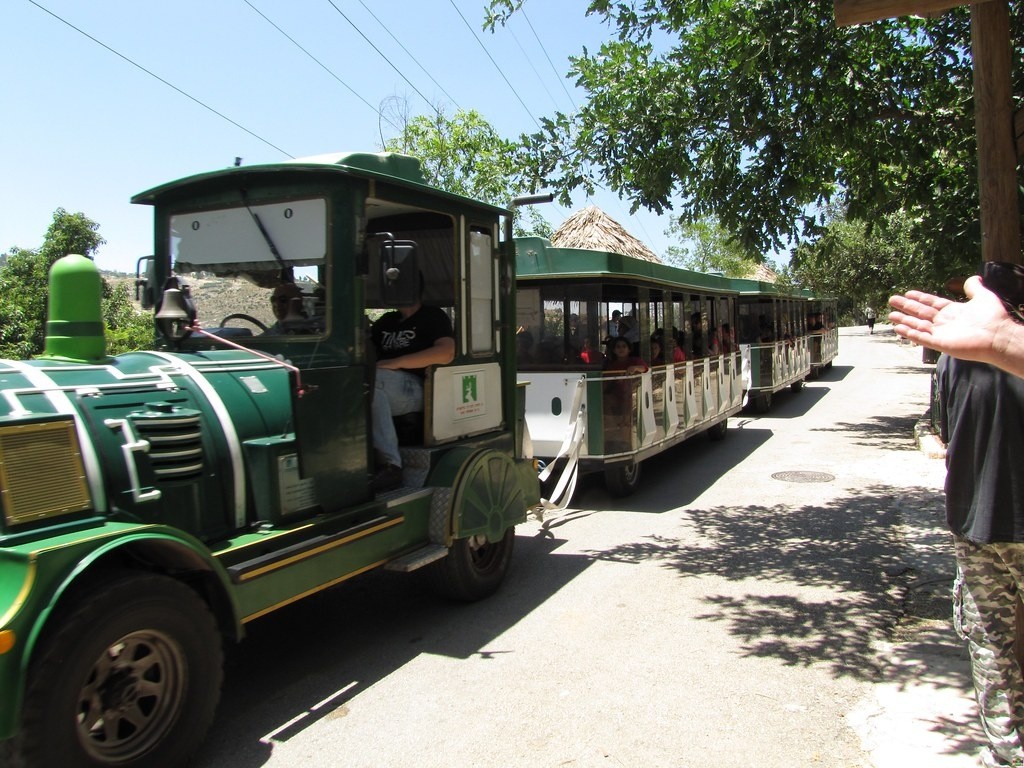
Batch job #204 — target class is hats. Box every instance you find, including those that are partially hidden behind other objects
[612,311,623,316]
[980,260,1024,306]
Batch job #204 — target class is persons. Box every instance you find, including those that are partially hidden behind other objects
[807,310,836,360]
[866,305,876,334]
[936,259,1024,768]
[888,276,1024,380]
[516,309,738,453]
[259,285,313,335]
[368,268,455,494]
[739,314,800,369]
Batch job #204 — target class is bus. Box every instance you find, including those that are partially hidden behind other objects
[0,152,851,767]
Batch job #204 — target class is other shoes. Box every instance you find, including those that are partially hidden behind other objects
[375,463,403,494]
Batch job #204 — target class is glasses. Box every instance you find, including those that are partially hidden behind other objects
[270,295,291,304]
